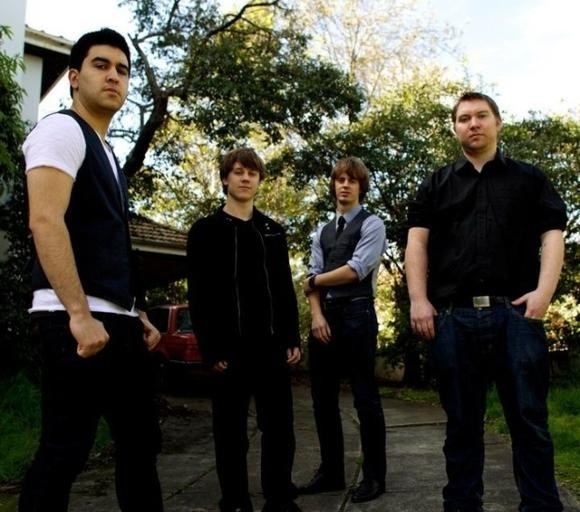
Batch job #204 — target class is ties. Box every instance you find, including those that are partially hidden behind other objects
[334,215,348,241]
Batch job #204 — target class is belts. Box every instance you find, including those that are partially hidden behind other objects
[444,291,519,313]
[323,293,371,313]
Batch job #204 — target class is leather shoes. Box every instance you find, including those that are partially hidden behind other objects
[351,480,388,503]
[298,475,346,493]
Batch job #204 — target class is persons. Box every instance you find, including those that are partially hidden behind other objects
[183,148,302,512]
[403,92,569,511]
[21,27,162,509]
[298,158,387,503]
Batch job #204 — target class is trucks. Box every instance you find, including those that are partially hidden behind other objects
[144,303,202,386]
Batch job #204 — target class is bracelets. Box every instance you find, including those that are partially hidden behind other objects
[309,274,317,289]
